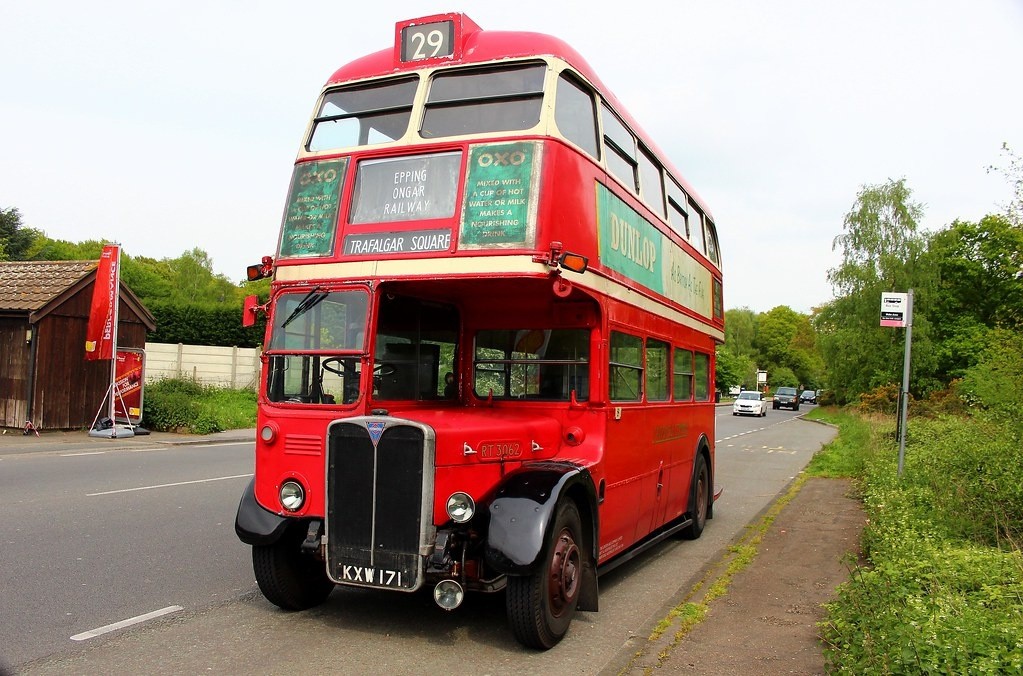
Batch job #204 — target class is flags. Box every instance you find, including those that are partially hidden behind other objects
[85,245,116,362]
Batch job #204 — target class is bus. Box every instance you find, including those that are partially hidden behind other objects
[234,10,726,653]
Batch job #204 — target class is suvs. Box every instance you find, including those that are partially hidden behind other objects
[772,386,803,410]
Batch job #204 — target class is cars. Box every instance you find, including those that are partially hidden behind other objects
[732,390,768,417]
[816,388,829,398]
[799,390,816,404]
